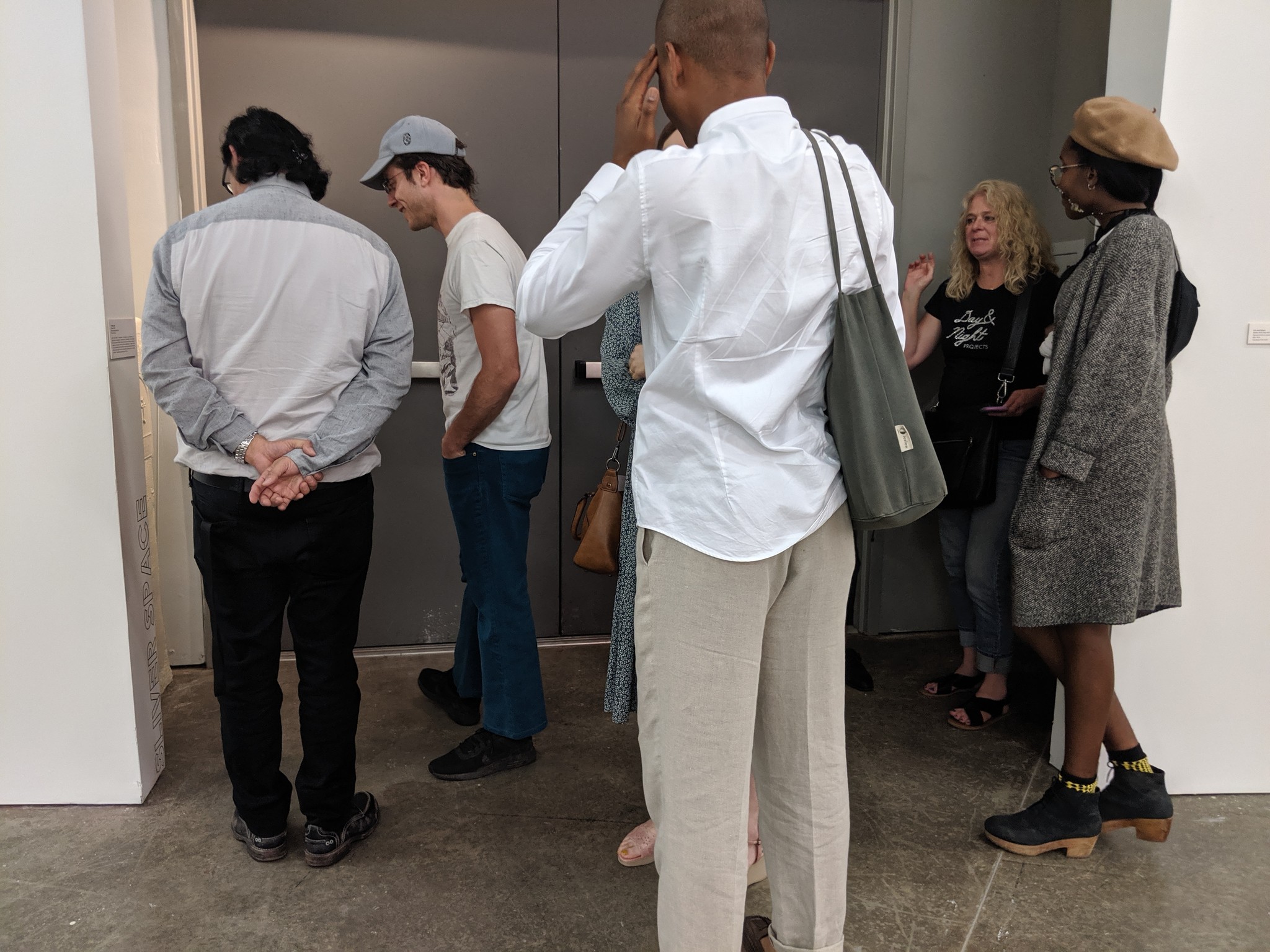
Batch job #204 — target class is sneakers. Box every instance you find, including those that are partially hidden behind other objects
[303,791,380,866]
[417,667,480,726]
[230,808,289,861]
[429,727,537,781]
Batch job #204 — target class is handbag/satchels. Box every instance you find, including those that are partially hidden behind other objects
[920,407,1001,514]
[570,418,626,575]
[801,126,948,532]
[1162,232,1200,369]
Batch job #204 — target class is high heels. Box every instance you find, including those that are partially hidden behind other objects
[983,776,1102,859]
[1098,766,1173,842]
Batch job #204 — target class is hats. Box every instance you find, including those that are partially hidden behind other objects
[1068,97,1179,172]
[359,116,466,190]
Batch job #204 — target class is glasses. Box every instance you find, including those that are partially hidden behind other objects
[1049,164,1085,187]
[382,164,432,194]
[222,163,234,195]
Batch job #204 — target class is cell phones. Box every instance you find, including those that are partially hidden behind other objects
[980,406,1006,412]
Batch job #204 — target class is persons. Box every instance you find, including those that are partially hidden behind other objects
[360,115,551,781]
[985,95,1184,859]
[600,123,770,887]
[140,106,415,867]
[901,179,1058,730]
[517,1,907,952]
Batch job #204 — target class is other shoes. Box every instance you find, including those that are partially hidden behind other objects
[743,915,772,952]
[845,649,874,692]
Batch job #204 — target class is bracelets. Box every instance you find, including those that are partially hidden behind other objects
[235,430,258,465]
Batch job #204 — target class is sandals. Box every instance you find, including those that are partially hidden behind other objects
[747,840,767,886]
[919,668,984,697]
[948,694,1012,730]
[618,819,654,867]
